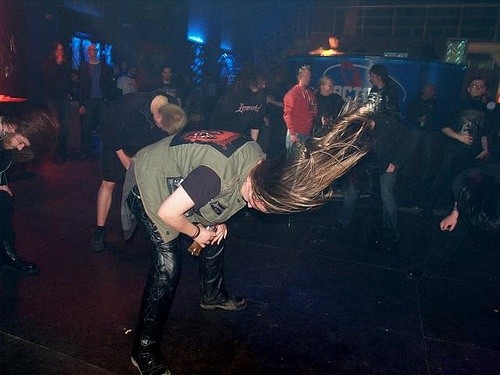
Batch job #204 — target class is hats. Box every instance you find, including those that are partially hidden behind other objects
[371,64,388,81]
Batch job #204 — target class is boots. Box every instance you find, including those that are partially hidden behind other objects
[0,239,39,275]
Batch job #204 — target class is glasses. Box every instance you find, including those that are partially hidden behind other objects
[470,84,485,89]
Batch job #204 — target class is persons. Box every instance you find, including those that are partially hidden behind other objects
[114,55,180,107]
[281,63,347,157]
[124,97,379,374]
[327,63,500,255]
[43,39,79,166]
[210,63,268,217]
[76,45,116,162]
[91,89,190,252]
[0,103,62,278]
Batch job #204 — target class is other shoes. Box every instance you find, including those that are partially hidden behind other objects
[80,153,87,161]
[90,226,105,251]
[375,236,398,246]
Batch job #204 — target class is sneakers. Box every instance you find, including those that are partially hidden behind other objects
[130,354,170,375]
[201,293,247,311]
